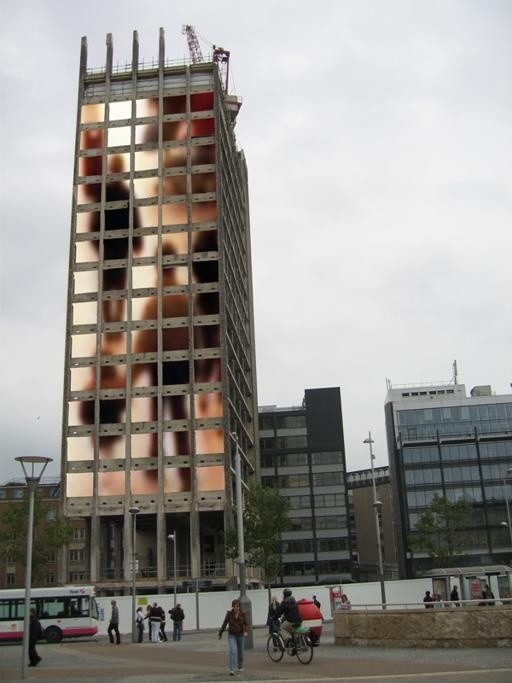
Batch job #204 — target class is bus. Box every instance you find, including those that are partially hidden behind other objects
[1,584,100,643]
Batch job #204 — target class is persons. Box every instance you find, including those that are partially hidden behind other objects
[266,596,280,651]
[341,593,351,610]
[268,588,302,648]
[28,607,42,666]
[218,599,248,675]
[72,601,78,614]
[422,584,495,608]
[136,602,185,643]
[312,595,321,608]
[107,599,120,644]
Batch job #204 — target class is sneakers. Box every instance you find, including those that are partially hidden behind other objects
[290,647,296,656]
[230,671,234,675]
[237,668,243,672]
[285,637,291,648]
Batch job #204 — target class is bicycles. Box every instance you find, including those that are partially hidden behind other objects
[263,615,314,666]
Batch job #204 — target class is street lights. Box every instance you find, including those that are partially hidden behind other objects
[15,454,54,682]
[128,505,140,642]
[501,467,512,540]
[362,430,387,609]
[167,529,178,607]
